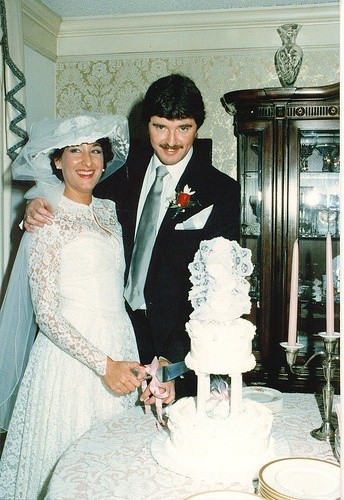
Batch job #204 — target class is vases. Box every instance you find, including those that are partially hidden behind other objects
[274,23,303,87]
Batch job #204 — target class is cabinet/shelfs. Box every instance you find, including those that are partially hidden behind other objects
[220,82,340,394]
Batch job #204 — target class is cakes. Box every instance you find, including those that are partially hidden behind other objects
[164,396,275,455]
[185,318,256,369]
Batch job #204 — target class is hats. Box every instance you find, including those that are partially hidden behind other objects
[12,112,131,195]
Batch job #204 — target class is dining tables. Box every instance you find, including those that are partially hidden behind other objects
[44,394,344,500]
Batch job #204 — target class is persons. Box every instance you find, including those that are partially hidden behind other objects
[23,73,243,400]
[0,112,149,500]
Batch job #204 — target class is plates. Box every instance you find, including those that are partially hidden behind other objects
[243,385,284,414]
[257,457,342,500]
[183,489,264,500]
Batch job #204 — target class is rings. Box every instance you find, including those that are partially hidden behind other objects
[25,215,30,219]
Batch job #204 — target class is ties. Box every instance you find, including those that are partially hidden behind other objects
[123,165,168,311]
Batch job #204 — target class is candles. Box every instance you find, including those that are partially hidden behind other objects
[325,231,335,333]
[288,240,299,346]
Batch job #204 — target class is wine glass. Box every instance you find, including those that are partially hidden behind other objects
[301,144,339,172]
[300,186,340,236]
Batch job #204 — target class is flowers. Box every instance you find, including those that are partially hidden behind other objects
[166,186,195,219]
